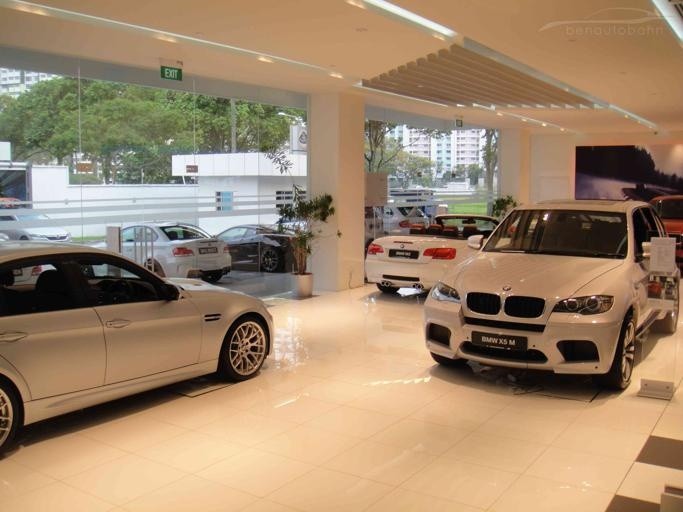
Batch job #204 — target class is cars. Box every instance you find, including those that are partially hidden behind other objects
[11,264,44,285]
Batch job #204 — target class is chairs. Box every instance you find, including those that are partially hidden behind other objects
[0,267,78,316]
[408,224,477,238]
[533,222,626,254]
[168,231,178,241]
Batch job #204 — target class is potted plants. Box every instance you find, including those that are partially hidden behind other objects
[278,191,343,299]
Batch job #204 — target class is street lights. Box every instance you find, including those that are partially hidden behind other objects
[278,112,303,127]
